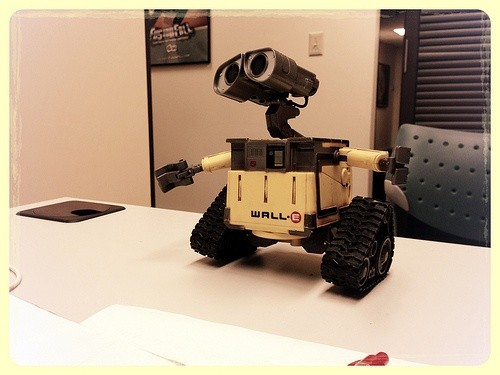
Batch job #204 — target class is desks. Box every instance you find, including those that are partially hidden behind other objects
[10,197,490,367]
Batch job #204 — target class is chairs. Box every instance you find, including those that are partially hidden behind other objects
[388,122,492,245]
